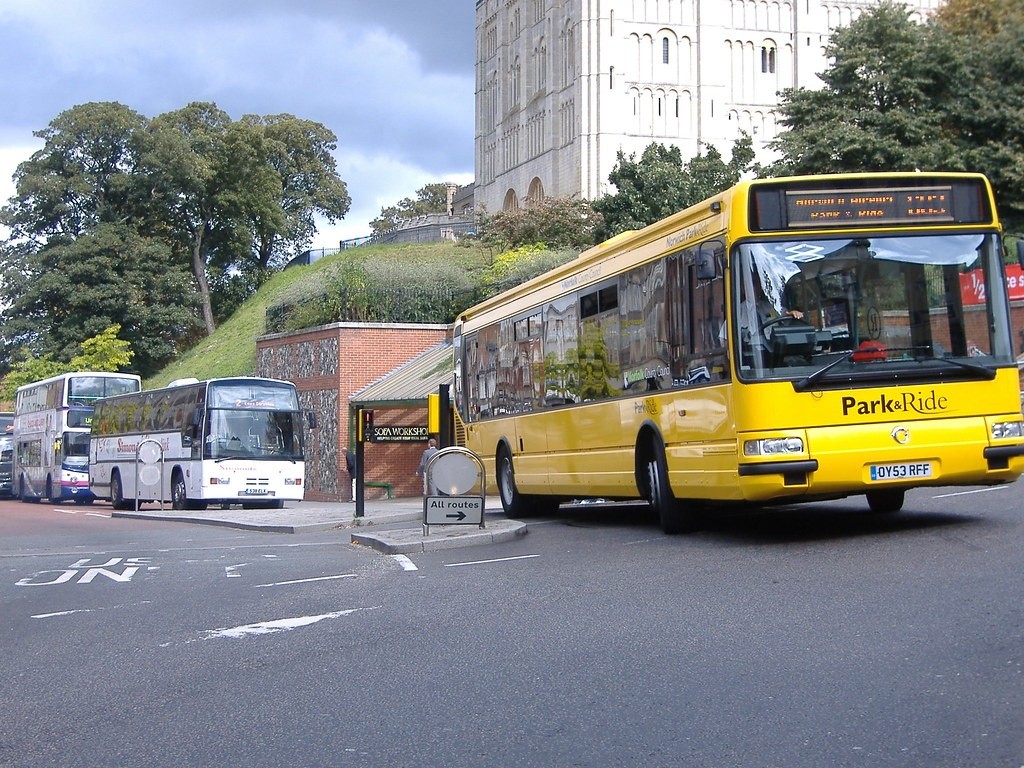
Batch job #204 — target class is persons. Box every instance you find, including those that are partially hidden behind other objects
[645,375,663,391]
[719,282,803,367]
[341,447,356,502]
[416,439,437,495]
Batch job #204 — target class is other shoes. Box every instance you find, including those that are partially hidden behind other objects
[349,500,356,502]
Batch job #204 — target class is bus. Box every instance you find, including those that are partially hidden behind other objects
[0,372,142,511]
[87,378,317,510]
[453,172,1024,534]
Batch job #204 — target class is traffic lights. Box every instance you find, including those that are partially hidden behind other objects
[359,408,374,442]
